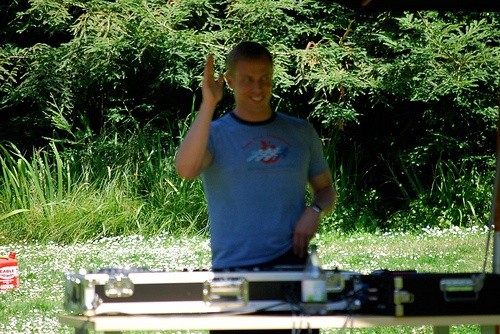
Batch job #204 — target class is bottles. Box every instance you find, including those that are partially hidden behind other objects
[302,244,327,313]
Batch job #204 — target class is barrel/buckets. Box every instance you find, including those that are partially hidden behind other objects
[0,251,19,290]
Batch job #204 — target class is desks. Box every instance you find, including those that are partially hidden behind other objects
[60,311,500,334]
